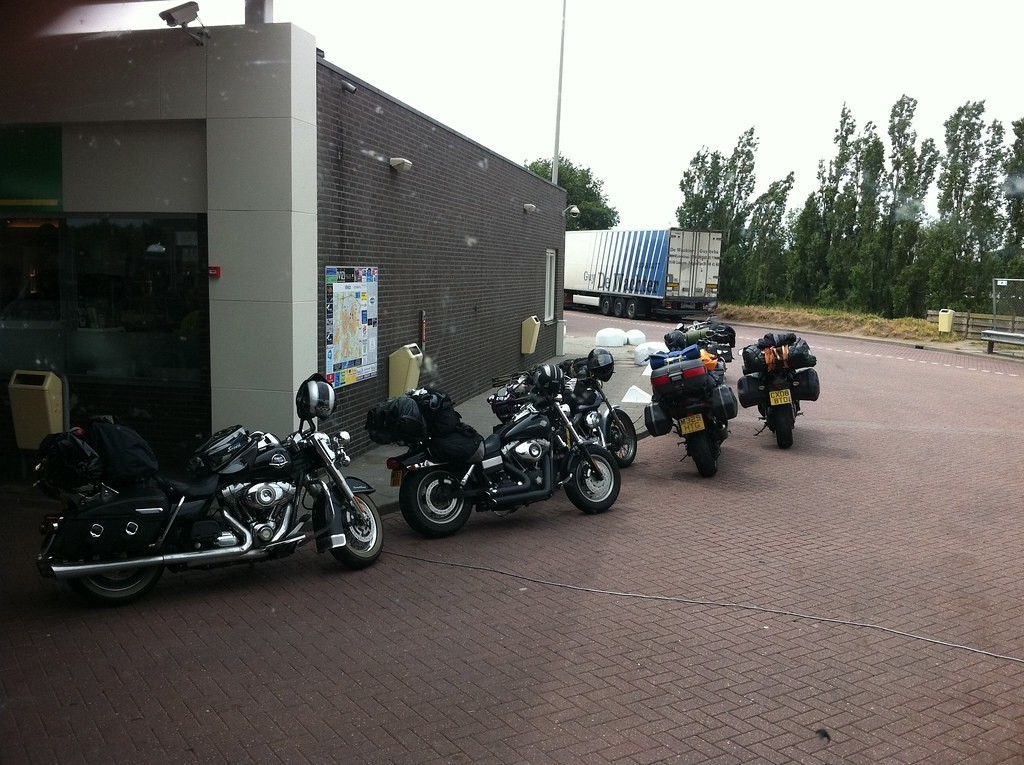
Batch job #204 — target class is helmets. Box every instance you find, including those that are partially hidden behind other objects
[295,372,337,421]
[588,348,614,382]
[534,364,565,390]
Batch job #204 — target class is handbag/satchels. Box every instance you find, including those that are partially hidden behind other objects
[708,324,735,348]
[79,414,157,488]
[29,430,102,495]
[407,386,456,436]
[431,423,486,466]
[743,332,817,376]
[648,345,726,391]
[366,398,426,445]
[185,424,250,474]
[663,332,685,352]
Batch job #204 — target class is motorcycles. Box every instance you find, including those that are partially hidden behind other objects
[486,356,638,491]
[366,362,621,540]
[32,413,384,608]
[738,334,820,449]
[644,300,738,477]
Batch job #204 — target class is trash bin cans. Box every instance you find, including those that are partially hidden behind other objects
[6,366,64,451]
[387,341,423,401]
[521,315,541,355]
[938,308,955,333]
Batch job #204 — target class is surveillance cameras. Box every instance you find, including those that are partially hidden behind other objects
[159,2,199,27]
[570,207,580,217]
